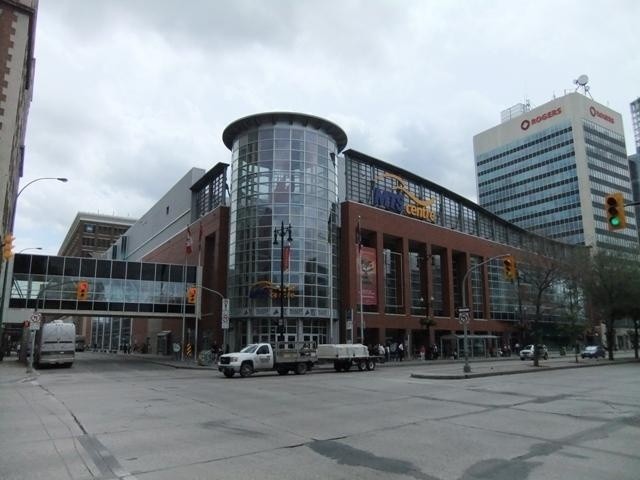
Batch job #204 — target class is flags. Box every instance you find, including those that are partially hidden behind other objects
[197,222,203,251]
[186,225,194,256]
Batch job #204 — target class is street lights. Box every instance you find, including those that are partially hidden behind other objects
[272,219,292,346]
[18,246,43,254]
[418,296,435,360]
[1,176,68,320]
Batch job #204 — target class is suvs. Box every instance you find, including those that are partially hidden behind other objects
[519,344,548,361]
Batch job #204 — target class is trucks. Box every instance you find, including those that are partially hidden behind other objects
[217,341,320,377]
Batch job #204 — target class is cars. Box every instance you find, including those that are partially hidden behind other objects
[581,345,605,359]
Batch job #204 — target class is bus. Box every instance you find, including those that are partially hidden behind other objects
[34,321,76,369]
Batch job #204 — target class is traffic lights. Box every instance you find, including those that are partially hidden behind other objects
[605,193,626,230]
[77,281,88,299]
[504,257,516,278]
[4,234,16,259]
[187,287,197,304]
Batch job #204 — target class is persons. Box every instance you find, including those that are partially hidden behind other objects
[370,340,521,362]
[211,340,220,364]
